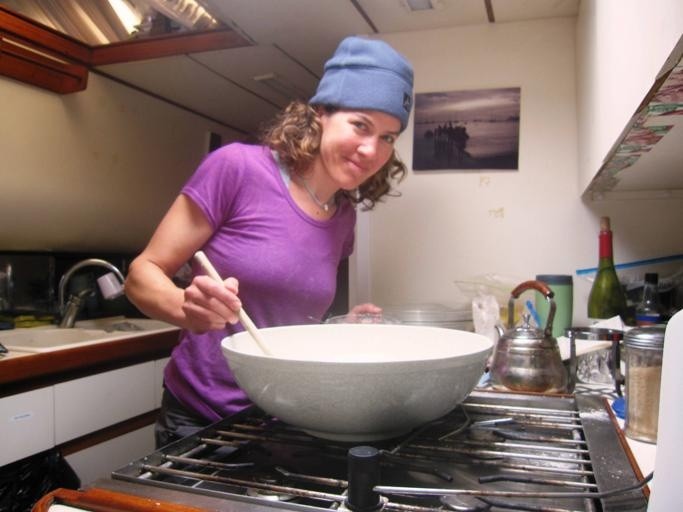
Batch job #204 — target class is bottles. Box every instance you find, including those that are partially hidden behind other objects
[623,328,664,445]
[587,217,664,329]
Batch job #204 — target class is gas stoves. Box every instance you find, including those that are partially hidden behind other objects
[99,388,655,512]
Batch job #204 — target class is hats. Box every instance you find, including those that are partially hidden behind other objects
[307,35,415,135]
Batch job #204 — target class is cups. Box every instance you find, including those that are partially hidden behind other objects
[535,273,572,341]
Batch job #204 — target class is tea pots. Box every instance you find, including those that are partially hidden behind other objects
[490,281,569,396]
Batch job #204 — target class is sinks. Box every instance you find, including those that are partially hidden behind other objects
[54,314,183,335]
[0,327,133,354]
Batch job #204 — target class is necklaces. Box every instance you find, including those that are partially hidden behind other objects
[296,169,340,214]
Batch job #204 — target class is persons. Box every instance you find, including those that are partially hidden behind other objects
[121,36,415,486]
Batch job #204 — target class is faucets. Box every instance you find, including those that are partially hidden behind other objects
[57,258,126,329]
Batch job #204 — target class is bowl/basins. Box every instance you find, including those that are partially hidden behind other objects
[219,324,496,443]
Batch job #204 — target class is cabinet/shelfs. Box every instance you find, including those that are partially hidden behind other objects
[0,355,174,489]
